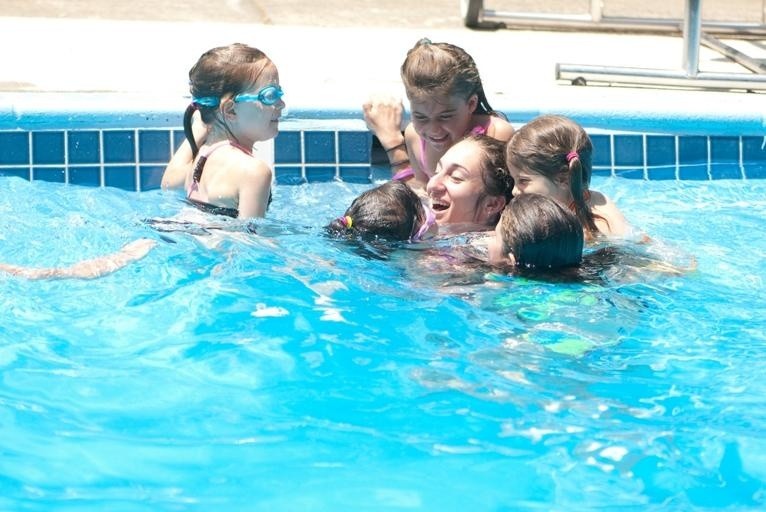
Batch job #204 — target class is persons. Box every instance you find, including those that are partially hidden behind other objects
[362,93,514,230]
[326,176,494,305]
[399,36,518,186]
[0,41,287,285]
[484,192,698,285]
[501,114,654,244]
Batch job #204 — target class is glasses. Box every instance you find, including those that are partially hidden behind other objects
[190,84,284,107]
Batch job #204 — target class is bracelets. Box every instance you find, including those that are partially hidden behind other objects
[392,168,414,180]
[390,158,410,168]
[384,142,406,154]
